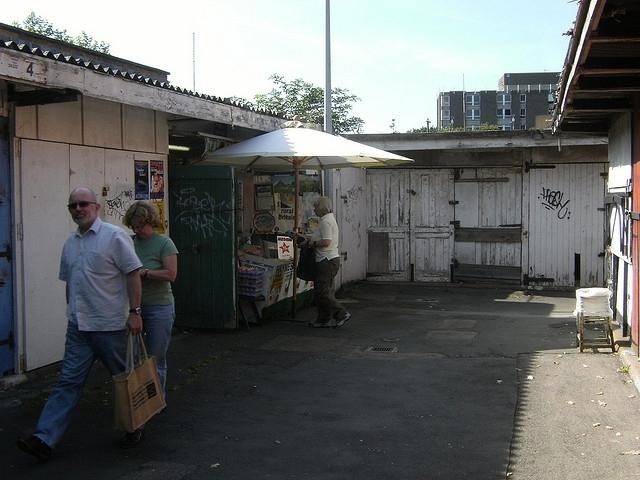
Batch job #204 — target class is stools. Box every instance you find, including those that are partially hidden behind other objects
[576,288,615,352]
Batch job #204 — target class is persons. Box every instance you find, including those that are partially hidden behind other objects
[13,184,146,467]
[119,198,180,411]
[294,197,352,332]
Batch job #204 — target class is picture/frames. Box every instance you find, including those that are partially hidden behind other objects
[254,183,275,211]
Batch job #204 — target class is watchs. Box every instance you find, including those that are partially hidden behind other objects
[144,268,149,278]
[128,307,142,316]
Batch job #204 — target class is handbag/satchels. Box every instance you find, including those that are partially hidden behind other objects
[294,238,318,282]
[112,326,169,434]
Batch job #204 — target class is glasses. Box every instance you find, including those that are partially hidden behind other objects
[64,200,98,211]
[128,223,148,231]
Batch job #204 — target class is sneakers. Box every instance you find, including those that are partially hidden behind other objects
[308,312,352,329]
[117,429,146,450]
[14,434,55,463]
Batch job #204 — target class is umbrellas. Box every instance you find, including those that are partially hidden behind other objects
[191,115,417,322]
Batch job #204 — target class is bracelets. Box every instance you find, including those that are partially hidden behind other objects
[313,240,317,248]
